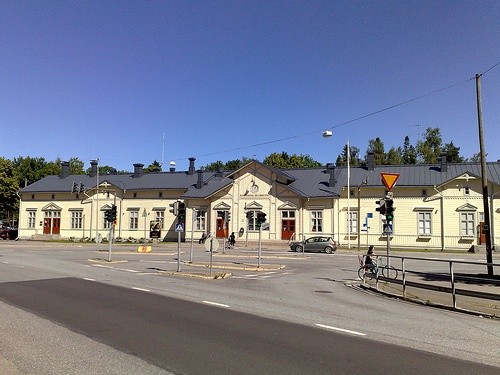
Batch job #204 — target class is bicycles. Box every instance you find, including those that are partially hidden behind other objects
[359,257,398,282]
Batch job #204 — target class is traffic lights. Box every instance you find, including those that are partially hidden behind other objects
[112,206,116,220]
[376,199,384,215]
[256,213,261,227]
[226,211,231,221]
[246,211,250,222]
[169,201,177,215]
[250,211,253,221]
[178,202,185,221]
[104,211,112,222]
[262,212,267,222]
[220,211,225,219]
[385,198,393,221]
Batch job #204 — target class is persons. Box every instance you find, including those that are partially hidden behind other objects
[365,245,379,274]
[229,232,235,247]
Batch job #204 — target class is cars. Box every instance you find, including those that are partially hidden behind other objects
[290,236,337,254]
